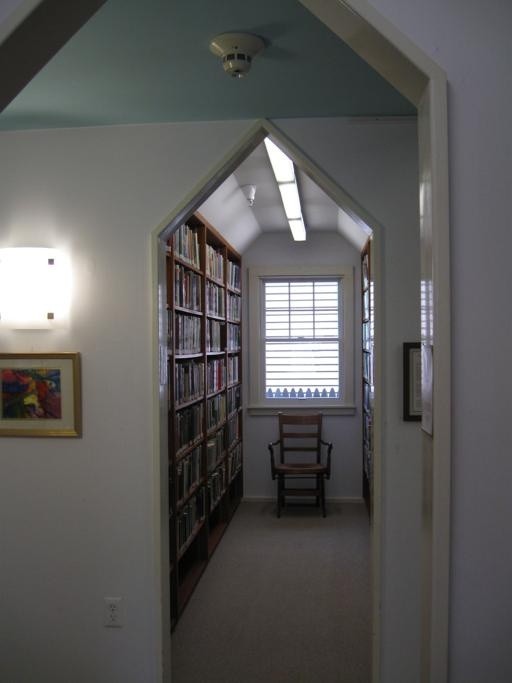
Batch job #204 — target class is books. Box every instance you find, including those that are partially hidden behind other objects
[362,254,371,481]
[166,223,243,550]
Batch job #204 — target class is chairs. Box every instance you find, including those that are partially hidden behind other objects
[268,412,332,519]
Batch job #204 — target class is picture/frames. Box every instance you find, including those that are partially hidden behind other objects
[0,352,83,438]
[403,342,421,421]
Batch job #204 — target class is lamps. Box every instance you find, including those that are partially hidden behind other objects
[0,246,71,330]
[263,137,307,242]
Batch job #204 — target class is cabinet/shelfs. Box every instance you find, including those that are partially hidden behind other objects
[166,209,244,634]
[359,238,372,524]
